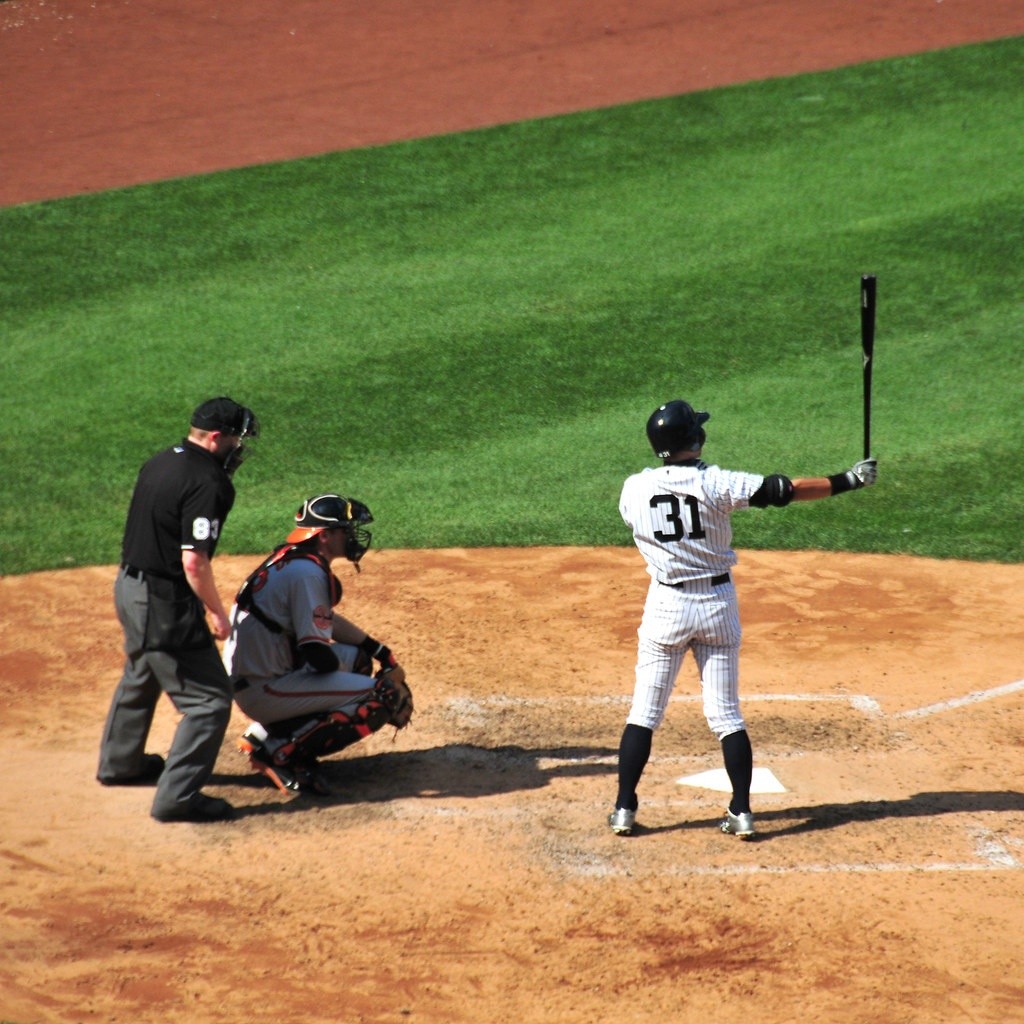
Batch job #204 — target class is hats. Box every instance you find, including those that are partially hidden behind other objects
[189,397,243,436]
[285,493,349,544]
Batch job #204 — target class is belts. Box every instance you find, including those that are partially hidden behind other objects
[659,572,732,588]
[118,559,139,580]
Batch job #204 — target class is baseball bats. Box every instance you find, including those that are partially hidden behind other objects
[860,275,878,458]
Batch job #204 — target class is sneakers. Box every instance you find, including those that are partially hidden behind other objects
[609,808,640,835]
[236,722,335,798]
[719,808,755,841]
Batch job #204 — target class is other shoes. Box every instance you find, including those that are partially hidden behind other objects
[98,753,236,820]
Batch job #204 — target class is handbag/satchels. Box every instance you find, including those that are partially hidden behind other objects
[142,575,214,651]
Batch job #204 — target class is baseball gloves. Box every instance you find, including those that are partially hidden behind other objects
[377,666,414,729]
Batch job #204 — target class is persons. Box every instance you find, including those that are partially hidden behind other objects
[220,494,411,797]
[607,399,881,848]
[93,391,262,821]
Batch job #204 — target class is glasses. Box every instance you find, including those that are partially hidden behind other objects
[330,524,353,534]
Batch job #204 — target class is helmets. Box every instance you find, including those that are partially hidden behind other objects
[646,400,710,458]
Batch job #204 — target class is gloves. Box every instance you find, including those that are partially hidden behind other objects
[846,456,879,489]
